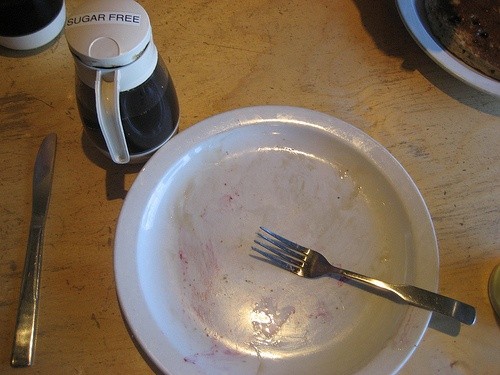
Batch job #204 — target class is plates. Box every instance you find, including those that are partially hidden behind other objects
[395,0,500,97]
[114,105,440,375]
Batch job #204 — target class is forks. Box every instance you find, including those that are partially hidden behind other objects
[250,227,477,325]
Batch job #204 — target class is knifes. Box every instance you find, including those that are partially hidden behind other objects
[13,134,57,364]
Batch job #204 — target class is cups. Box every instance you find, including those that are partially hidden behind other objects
[0,0,66,50]
[65,0,180,163]
